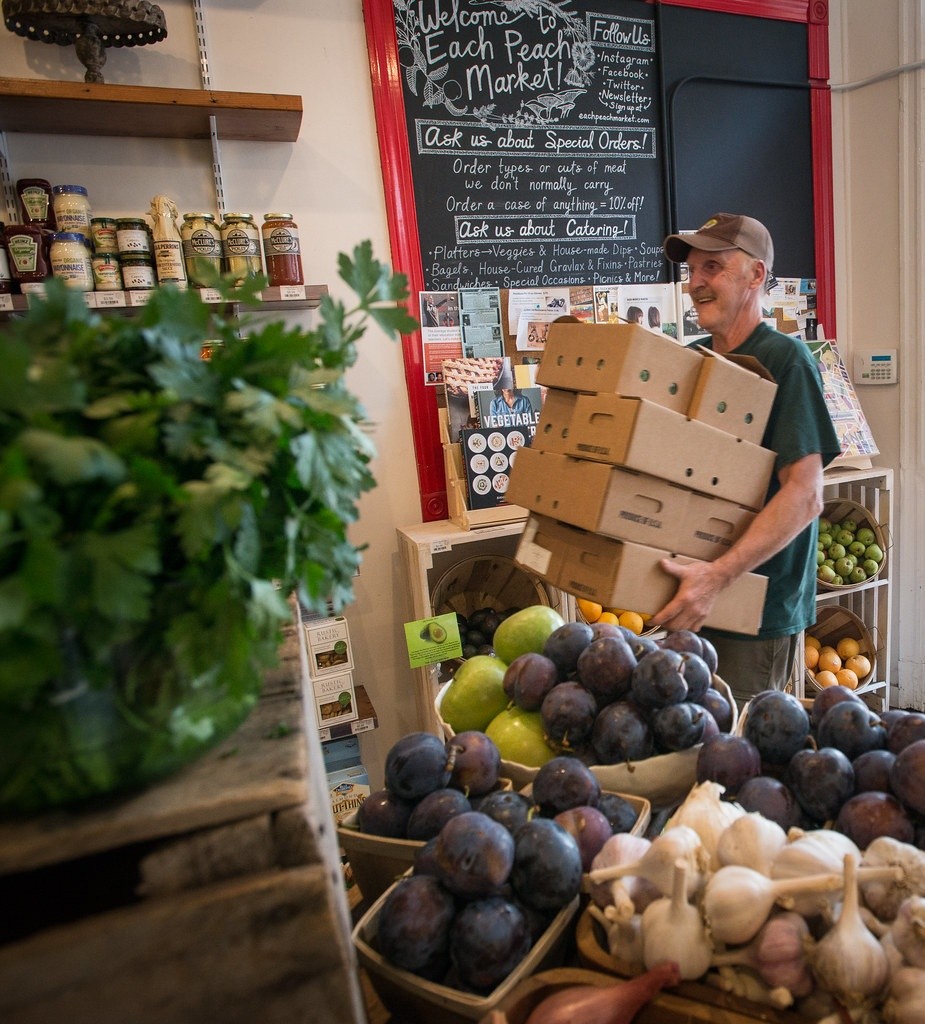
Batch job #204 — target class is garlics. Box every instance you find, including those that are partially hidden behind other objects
[524,782,925,1024]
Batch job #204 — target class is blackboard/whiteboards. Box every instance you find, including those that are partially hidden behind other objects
[362,1,836,288]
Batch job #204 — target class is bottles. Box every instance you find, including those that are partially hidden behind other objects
[154,195,188,292]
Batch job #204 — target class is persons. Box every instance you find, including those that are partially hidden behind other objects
[787,285,795,294]
[595,291,607,321]
[628,306,661,333]
[646,214,842,713]
[490,389,533,427]
[425,294,454,327]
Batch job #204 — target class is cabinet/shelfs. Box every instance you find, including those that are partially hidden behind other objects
[798,467,895,713]
[0,77,329,322]
[395,465,897,745]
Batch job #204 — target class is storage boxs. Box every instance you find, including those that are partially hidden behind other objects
[301,601,378,858]
[336,776,512,908]
[480,967,764,1024]
[352,781,651,1024]
[575,698,814,1024]
[504,313,779,637]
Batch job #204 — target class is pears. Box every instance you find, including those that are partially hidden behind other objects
[817,519,883,585]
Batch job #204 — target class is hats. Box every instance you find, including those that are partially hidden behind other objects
[663,212,775,273]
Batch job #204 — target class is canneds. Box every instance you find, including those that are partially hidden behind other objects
[0,178,309,291]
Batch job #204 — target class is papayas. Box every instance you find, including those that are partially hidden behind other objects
[438,607,564,765]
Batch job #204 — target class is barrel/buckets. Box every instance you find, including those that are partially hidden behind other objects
[431,555,560,671]
[802,605,886,698]
[815,499,893,592]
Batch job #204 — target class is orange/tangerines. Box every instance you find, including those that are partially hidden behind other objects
[575,598,654,634]
[804,636,871,689]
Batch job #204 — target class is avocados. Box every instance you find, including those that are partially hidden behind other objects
[421,621,447,642]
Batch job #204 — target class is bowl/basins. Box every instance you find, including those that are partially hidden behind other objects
[432,673,738,813]
[352,782,651,1019]
[337,778,513,904]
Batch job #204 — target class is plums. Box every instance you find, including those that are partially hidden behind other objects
[696,686,925,851]
[503,622,732,766]
[358,733,637,987]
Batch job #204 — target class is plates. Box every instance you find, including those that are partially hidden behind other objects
[467,431,524,496]
